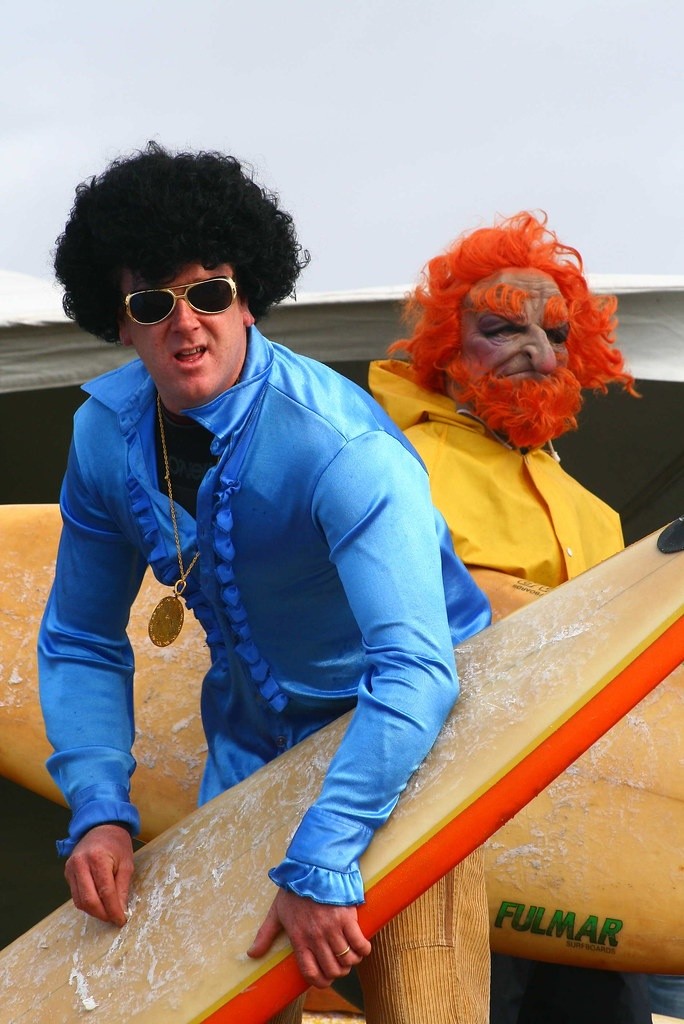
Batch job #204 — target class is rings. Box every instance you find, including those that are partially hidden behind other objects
[335,945,351,957]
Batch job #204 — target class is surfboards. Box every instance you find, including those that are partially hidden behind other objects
[0,504,684,1024]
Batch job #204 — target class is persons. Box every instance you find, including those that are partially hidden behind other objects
[37,139,495,1011]
[367,208,684,1024]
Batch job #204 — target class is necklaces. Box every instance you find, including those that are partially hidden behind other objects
[148,393,199,649]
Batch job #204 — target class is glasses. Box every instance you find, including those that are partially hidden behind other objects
[120,274,242,325]
